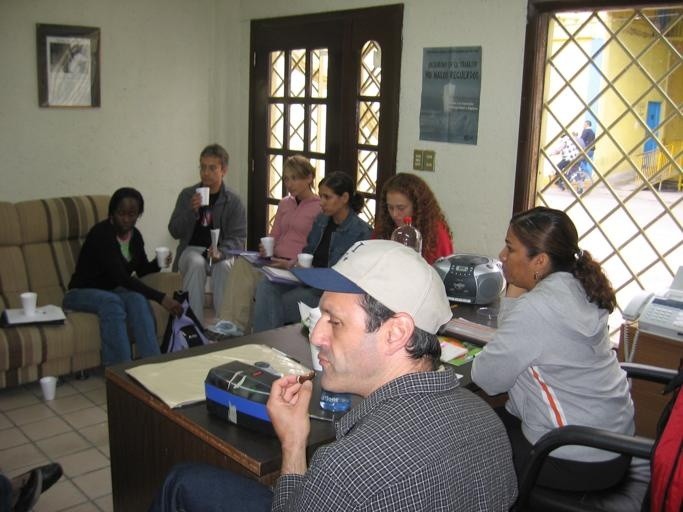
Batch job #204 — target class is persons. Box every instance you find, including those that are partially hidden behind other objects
[168,144,247,328]
[148,238,520,512]
[371,173,453,266]
[252,170,373,333]
[470,206,636,492]
[203,155,323,342]
[547,120,595,194]
[62,187,184,369]
[0,462,63,512]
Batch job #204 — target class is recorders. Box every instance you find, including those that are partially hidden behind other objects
[433,254,507,305]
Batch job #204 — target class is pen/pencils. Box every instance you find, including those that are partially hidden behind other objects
[466,351,482,360]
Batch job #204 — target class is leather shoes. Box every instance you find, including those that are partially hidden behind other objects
[11,462,63,512]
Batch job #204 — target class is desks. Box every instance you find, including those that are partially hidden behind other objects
[104,296,524,512]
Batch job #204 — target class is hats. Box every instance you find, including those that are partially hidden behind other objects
[288,239,453,336]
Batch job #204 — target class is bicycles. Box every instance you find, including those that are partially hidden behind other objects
[544,152,595,198]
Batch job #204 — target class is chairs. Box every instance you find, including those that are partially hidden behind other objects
[510,362,683,512]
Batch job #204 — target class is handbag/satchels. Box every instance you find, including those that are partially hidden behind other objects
[168,299,210,352]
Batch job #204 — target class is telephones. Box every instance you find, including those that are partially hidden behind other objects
[623,288,683,340]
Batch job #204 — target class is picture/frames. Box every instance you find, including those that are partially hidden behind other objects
[34,23,102,109]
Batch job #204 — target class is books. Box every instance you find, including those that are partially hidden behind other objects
[225,248,301,285]
[125,344,317,409]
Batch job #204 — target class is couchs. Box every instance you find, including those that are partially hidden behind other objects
[0,195,182,392]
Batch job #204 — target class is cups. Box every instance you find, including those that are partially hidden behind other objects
[195,187,210,207]
[40,376,57,402]
[20,292,38,317]
[154,246,170,269]
[259,236,275,258]
[296,253,313,269]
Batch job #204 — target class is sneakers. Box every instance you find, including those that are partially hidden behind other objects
[204,319,244,341]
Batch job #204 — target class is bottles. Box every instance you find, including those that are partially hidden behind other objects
[390,216,422,257]
[317,388,352,412]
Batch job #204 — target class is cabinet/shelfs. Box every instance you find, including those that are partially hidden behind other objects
[617,321,683,439]
